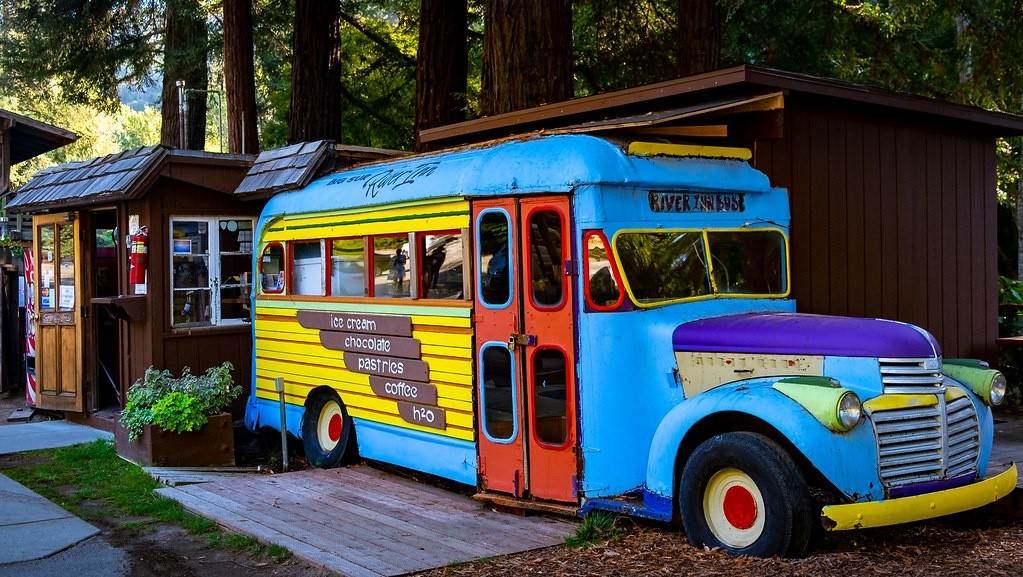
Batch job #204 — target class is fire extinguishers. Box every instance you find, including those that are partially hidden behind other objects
[129,226,149,284]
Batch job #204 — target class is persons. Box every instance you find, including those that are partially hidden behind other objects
[426,244,445,290]
[389,248,406,291]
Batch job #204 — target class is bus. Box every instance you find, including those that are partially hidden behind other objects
[246,130,1021,558]
